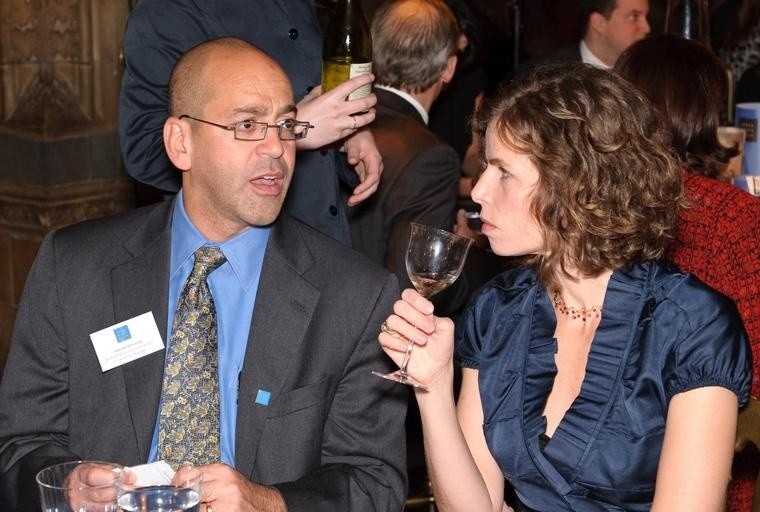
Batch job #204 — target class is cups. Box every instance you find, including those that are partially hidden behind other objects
[33,454,206,512]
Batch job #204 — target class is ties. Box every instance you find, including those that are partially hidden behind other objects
[157,246,228,473]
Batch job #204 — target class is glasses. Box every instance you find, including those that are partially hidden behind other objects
[178,113,315,142]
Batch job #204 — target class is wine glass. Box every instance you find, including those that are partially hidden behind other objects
[370,220,475,392]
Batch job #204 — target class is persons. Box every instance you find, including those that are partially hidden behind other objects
[1,37,409,512]
[334,2,759,400]
[120,0,384,247]
[378,65,751,510]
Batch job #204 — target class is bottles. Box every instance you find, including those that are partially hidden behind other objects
[321,1,371,135]
